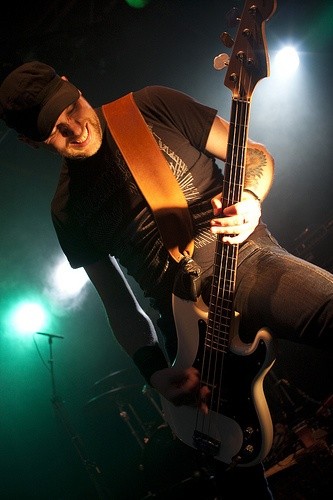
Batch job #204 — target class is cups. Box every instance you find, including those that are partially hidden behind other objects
[291,420,317,448]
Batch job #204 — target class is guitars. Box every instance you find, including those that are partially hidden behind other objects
[161,1,283,468]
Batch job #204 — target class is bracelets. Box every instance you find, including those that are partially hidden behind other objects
[243,188,261,203]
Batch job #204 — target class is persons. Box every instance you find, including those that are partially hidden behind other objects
[263,393,333,489]
[0,62,333,500]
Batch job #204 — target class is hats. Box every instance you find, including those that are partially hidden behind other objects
[0,60,81,146]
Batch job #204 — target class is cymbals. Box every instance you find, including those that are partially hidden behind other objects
[80,384,136,413]
[92,365,132,392]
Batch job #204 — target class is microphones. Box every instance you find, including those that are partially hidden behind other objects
[37,332,65,339]
